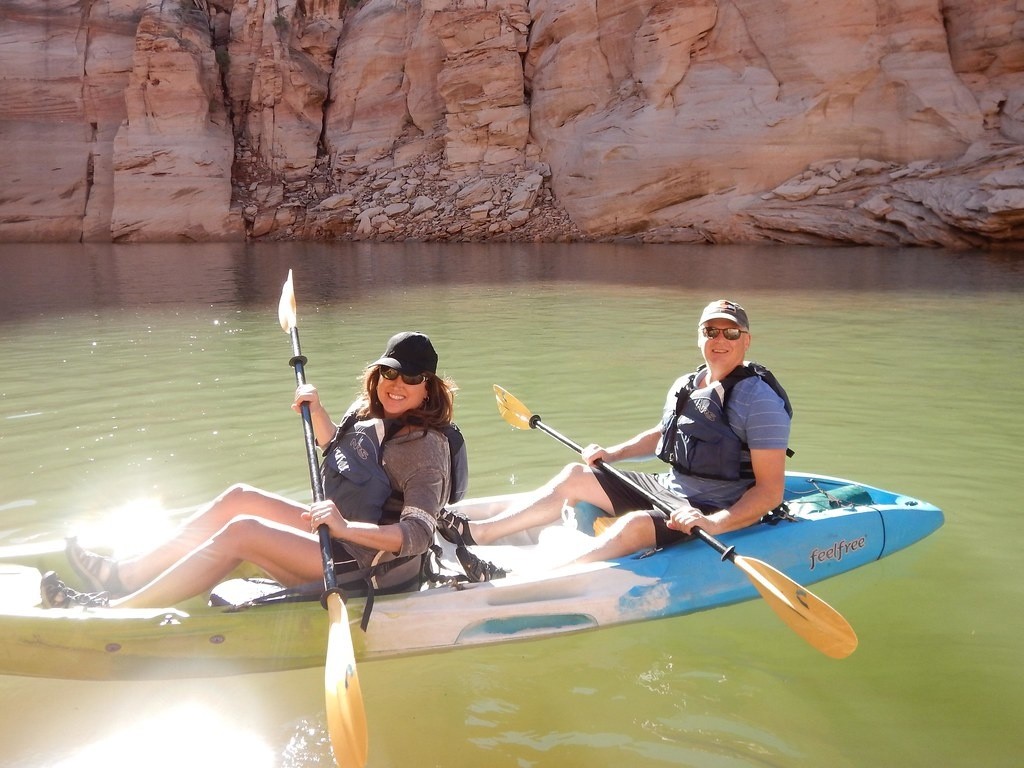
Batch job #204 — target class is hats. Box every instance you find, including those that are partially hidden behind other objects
[369,331,438,376]
[699,299,749,331]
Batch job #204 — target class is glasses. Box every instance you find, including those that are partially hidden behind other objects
[702,326,746,340]
[378,364,430,385]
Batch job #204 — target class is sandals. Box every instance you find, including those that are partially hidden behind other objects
[437,507,478,548]
[66,538,137,599]
[41,571,110,610]
[456,546,511,583]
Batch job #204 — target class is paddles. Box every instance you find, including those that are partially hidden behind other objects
[491,382,859,662]
[277,266,371,768]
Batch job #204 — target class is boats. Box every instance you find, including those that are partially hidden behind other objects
[0,469,946,680]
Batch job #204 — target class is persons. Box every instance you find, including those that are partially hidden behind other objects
[436,299,793,582]
[42,331,468,608]
